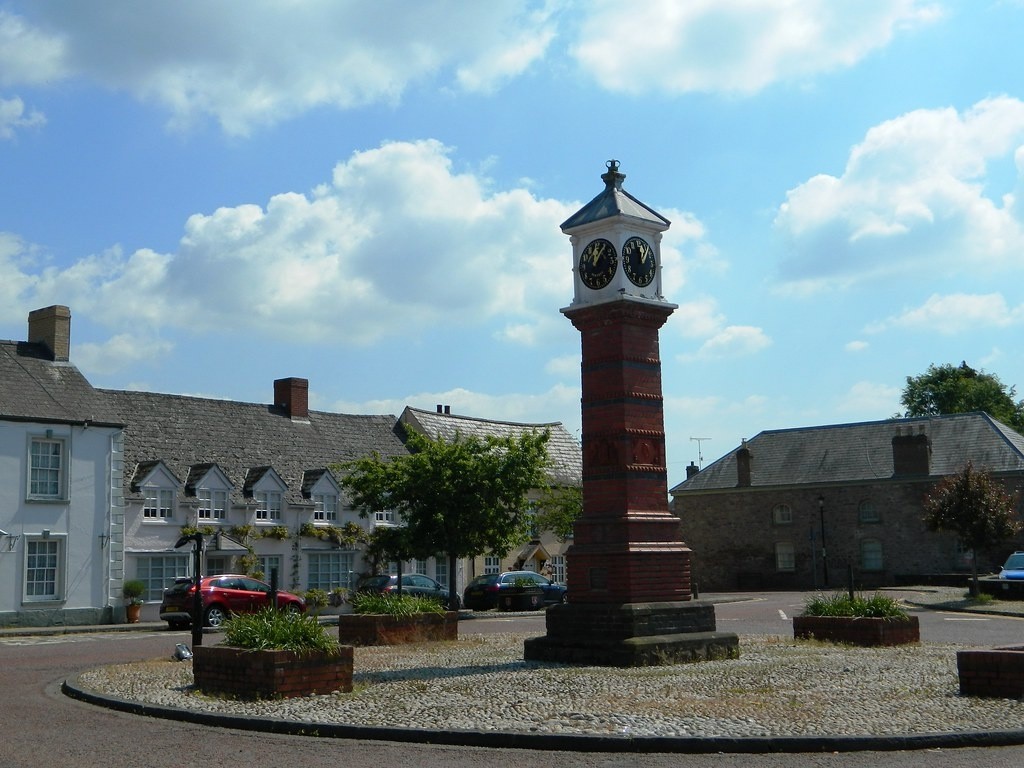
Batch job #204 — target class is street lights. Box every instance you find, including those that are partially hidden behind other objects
[817,494,827,586]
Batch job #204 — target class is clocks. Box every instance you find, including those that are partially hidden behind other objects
[579,239,618,290]
[622,237,656,287]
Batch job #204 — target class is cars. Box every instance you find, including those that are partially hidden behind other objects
[463,571,568,607]
[361,573,461,609]
[159,575,307,627]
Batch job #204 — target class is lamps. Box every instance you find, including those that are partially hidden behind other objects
[171,643,194,662]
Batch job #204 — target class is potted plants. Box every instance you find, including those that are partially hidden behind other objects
[122,579,145,623]
[497,574,544,612]
[192,605,354,701]
[339,592,459,646]
[792,591,920,647]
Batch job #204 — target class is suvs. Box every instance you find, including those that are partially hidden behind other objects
[998,551,1024,580]
